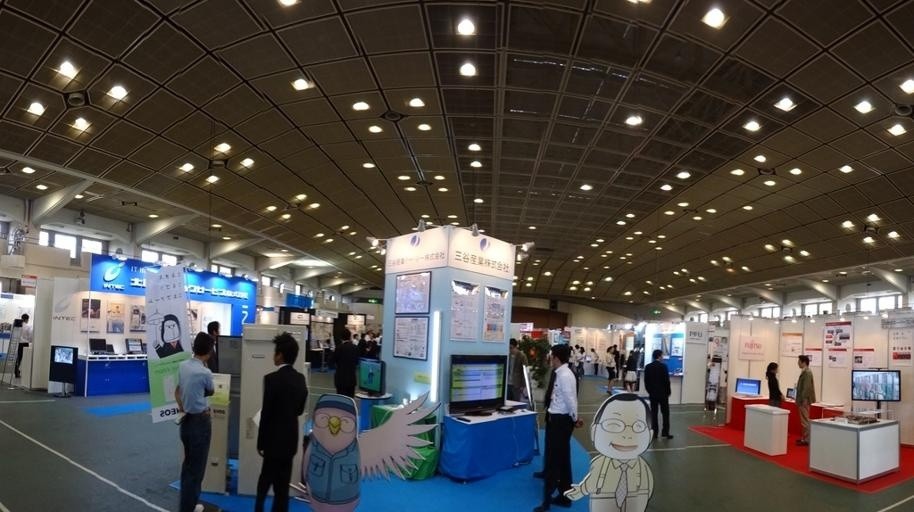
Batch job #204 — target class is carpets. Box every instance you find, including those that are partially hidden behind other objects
[169,429,592,512]
[689,423,914,494]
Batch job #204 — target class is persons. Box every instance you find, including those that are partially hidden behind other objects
[153,314,183,359]
[795,355,817,446]
[506,338,528,402]
[174,332,216,512]
[644,348,675,440]
[517,336,552,389]
[351,331,383,360]
[563,395,656,512]
[766,361,789,406]
[331,328,359,400]
[605,344,637,396]
[534,344,586,510]
[254,330,308,512]
[591,349,599,376]
[14,314,32,377]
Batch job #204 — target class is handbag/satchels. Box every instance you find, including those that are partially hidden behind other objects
[625,371,637,381]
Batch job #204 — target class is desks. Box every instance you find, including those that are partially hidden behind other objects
[437,405,538,482]
[744,404,790,456]
[730,394,846,437]
[808,419,901,484]
[583,362,617,379]
[356,392,392,431]
[371,403,439,479]
[310,348,335,369]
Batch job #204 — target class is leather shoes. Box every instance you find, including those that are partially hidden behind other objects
[654,434,673,438]
[534,495,571,511]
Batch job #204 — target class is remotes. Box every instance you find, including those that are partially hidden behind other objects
[458,417,471,422]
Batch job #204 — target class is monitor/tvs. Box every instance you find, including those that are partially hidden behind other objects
[355,356,387,397]
[734,377,761,397]
[786,387,794,398]
[851,370,902,402]
[126,338,145,354]
[89,338,108,355]
[448,354,510,416]
[107,344,118,355]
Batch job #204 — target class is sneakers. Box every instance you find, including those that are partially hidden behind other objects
[607,391,612,395]
[796,439,809,445]
[534,472,544,478]
[193,504,204,512]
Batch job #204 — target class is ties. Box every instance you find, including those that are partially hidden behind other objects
[615,463,628,508]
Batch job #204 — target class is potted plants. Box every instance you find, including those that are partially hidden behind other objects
[520,337,552,403]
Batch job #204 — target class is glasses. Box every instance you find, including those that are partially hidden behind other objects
[595,418,650,432]
